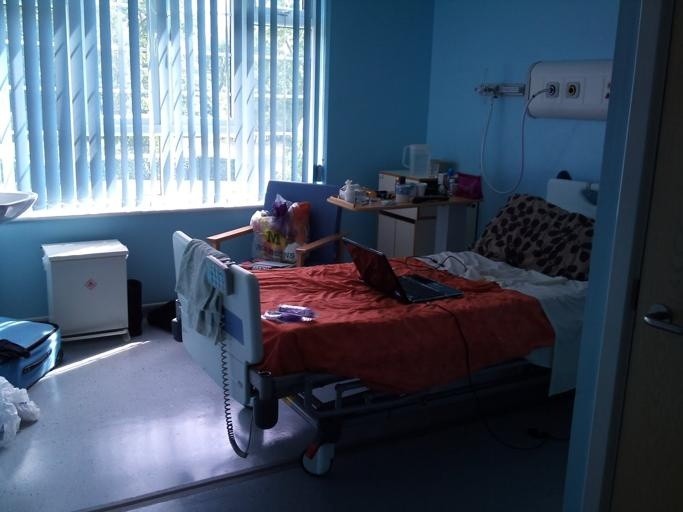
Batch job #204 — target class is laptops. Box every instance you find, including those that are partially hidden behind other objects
[341,236,464,305]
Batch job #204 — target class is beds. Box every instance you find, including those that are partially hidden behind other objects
[170,176,603,477]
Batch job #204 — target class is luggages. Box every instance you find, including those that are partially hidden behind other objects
[0,315,64,389]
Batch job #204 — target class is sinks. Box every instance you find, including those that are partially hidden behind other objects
[1,191,38,221]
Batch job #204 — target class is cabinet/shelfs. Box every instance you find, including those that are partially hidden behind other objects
[376,170,481,263]
[43,240,132,348]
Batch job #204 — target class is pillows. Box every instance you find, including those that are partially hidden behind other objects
[469,190,599,281]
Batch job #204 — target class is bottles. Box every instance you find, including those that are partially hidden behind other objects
[448,179,455,195]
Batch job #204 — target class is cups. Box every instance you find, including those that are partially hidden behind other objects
[401,143,433,178]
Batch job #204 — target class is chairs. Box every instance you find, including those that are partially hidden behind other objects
[205,179,344,270]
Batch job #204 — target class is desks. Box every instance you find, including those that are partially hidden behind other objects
[329,188,482,241]
